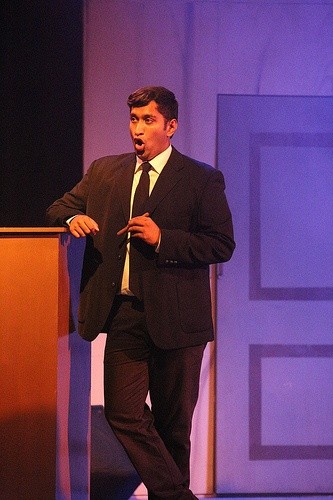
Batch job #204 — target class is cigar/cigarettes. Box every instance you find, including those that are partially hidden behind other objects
[116,212,149,237]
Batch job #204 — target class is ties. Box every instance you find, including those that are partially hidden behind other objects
[129,162,153,307]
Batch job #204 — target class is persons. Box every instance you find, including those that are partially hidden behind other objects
[46,86,237,500]
